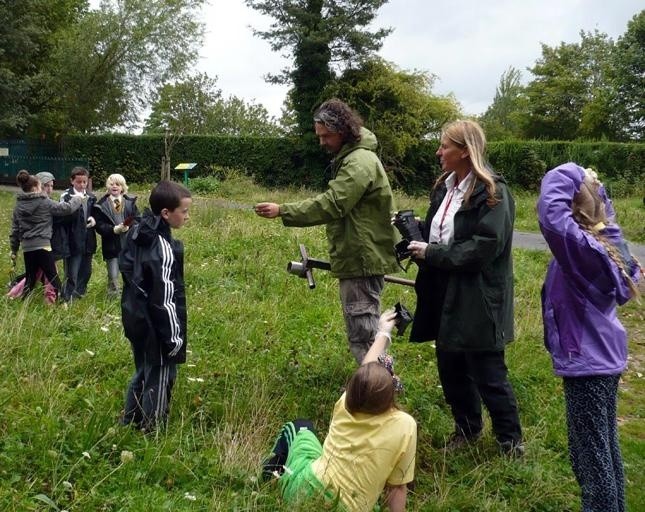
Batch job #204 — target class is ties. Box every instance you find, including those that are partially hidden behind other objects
[114,199,120,212]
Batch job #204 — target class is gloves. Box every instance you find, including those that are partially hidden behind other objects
[114,223,129,234]
[86,216,96,228]
[375,307,397,343]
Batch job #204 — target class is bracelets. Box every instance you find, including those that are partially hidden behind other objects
[373,331,392,344]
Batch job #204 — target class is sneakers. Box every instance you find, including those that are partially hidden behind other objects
[498,440,524,459]
[439,432,480,449]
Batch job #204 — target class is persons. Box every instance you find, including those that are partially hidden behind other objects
[7,172,58,304]
[391,119,524,458]
[93,174,141,294]
[59,166,96,302]
[10,170,82,301]
[123,181,192,434]
[263,306,417,512]
[536,162,645,512]
[255,97,401,392]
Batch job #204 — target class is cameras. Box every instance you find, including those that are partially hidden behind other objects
[388,209,425,272]
